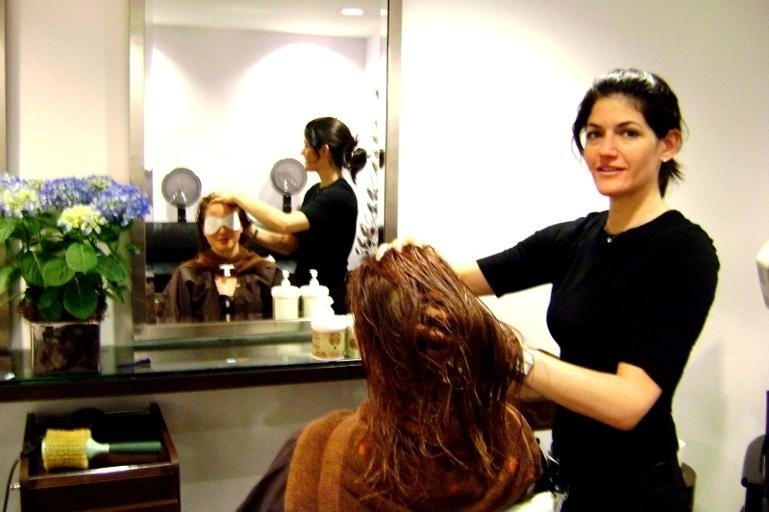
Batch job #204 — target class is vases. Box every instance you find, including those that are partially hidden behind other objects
[28,317,107,376]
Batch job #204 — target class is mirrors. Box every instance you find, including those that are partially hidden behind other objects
[127,11,401,353]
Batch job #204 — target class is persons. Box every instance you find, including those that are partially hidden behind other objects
[262,247,548,512]
[208,116,367,315]
[376,66,721,512]
[151,194,282,320]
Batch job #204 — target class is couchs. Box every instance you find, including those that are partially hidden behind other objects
[146,222,268,294]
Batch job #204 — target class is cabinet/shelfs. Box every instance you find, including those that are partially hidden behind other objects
[20,405,185,512]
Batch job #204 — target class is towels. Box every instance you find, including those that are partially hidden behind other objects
[281,393,543,511]
[179,247,282,274]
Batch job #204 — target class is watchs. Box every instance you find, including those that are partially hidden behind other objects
[506,343,537,385]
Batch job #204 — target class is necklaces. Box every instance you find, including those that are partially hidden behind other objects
[594,212,663,244]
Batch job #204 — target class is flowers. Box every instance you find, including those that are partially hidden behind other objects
[1,172,152,324]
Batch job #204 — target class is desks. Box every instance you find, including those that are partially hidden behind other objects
[0,329,485,512]
